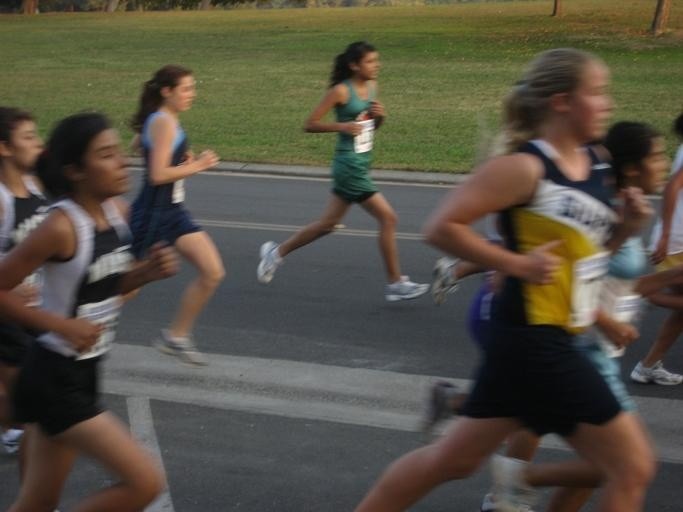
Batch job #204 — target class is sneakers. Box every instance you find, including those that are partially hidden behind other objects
[429,254,463,307]
[420,379,461,436]
[256,239,283,285]
[631,360,683,386]
[0,428,28,457]
[481,450,536,511]
[154,327,209,370]
[383,275,430,303]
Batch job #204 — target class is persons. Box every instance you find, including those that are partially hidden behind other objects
[118,63,225,366]
[0,107,50,454]
[257,42,432,303]
[0,115,179,512]
